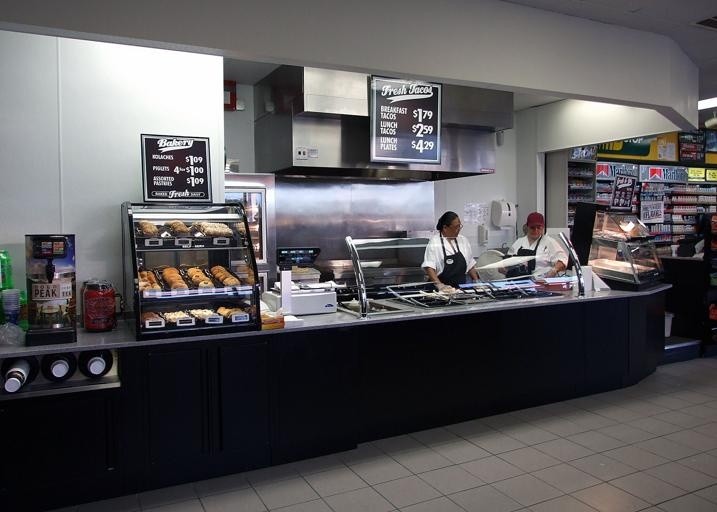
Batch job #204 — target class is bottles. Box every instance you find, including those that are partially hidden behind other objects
[19,290,29,330]
[1,249,15,289]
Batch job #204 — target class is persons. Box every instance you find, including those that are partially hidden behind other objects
[420,211,479,293]
[496,211,569,279]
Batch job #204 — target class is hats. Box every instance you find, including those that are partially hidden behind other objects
[528,212,544,228]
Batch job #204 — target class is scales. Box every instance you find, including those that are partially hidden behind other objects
[261,281,337,316]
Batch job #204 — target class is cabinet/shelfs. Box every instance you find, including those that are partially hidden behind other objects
[545,149,717,292]
[119,199,262,342]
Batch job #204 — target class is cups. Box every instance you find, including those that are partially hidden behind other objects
[2,289,19,324]
[4,367,27,393]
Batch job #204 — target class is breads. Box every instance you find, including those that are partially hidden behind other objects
[137,220,245,326]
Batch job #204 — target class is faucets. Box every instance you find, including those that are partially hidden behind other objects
[558,230,585,296]
[343,235,368,319]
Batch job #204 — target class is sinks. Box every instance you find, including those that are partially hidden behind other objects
[337,298,415,315]
